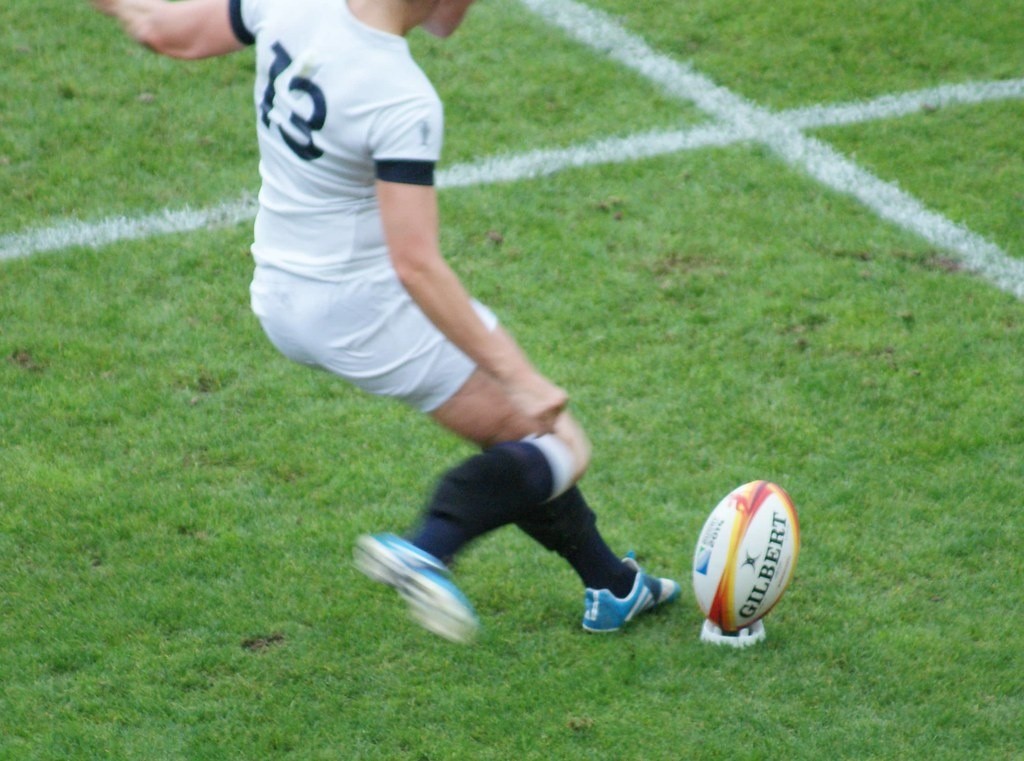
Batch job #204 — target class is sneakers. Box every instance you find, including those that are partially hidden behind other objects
[351,530,480,640]
[580,551,683,635]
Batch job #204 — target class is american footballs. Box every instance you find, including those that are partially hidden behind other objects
[691,480,800,632]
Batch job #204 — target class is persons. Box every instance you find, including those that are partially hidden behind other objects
[85,0,684,642]
